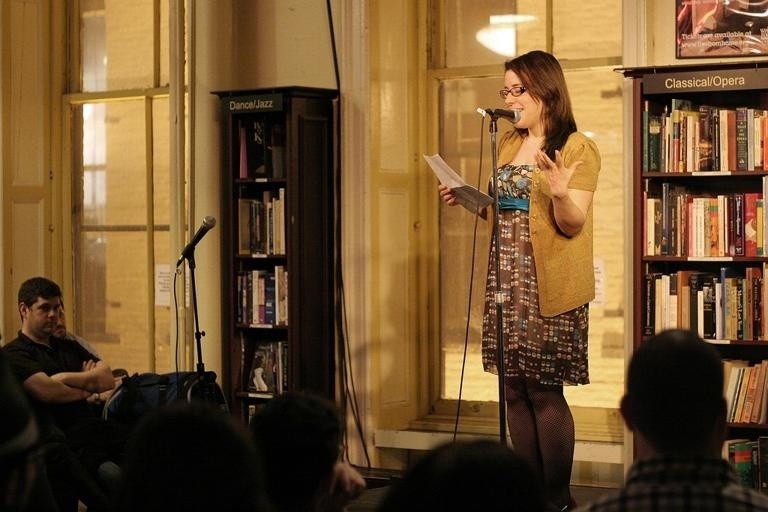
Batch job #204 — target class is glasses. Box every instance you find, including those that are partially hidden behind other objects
[497,86,527,99]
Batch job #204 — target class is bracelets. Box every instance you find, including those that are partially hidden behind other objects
[95,390,100,403]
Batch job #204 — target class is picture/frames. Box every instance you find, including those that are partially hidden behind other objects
[675,0,768,58]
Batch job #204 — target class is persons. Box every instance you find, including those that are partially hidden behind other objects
[373,436,556,510]
[250,392,366,511]
[2,278,116,427]
[52,298,128,404]
[436,49,603,511]
[0,354,111,512]
[572,327,767,510]
[115,400,274,511]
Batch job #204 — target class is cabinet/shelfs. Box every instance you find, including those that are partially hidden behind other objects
[210,85,340,426]
[625,63,768,467]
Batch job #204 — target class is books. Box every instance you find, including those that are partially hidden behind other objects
[234,113,290,424]
[716,355,767,495]
[640,98,766,341]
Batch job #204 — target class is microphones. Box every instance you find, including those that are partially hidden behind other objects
[176,215,216,268]
[485,108,521,123]
